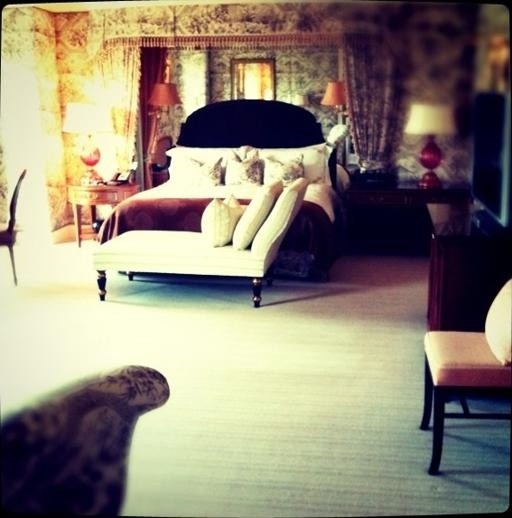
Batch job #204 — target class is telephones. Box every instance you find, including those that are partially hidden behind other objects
[111,169,131,181]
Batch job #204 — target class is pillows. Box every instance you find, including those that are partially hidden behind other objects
[201,194,244,248]
[326,146,334,187]
[189,157,223,185]
[225,150,263,186]
[232,178,284,249]
[165,146,248,184]
[263,154,303,188]
[257,142,326,185]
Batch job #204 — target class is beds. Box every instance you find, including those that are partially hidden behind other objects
[97,98,337,282]
[96,178,310,308]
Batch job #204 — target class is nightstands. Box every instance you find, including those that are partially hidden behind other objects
[67,184,139,247]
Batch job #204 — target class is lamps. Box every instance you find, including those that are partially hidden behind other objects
[60,103,113,166]
[319,82,353,116]
[147,82,183,119]
[404,103,458,187]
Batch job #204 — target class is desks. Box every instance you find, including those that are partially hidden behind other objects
[427,235,484,333]
[338,186,458,258]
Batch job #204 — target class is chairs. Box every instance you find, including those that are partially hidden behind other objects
[420,279,512,476]
[1,170,29,287]
[1,365,170,516]
